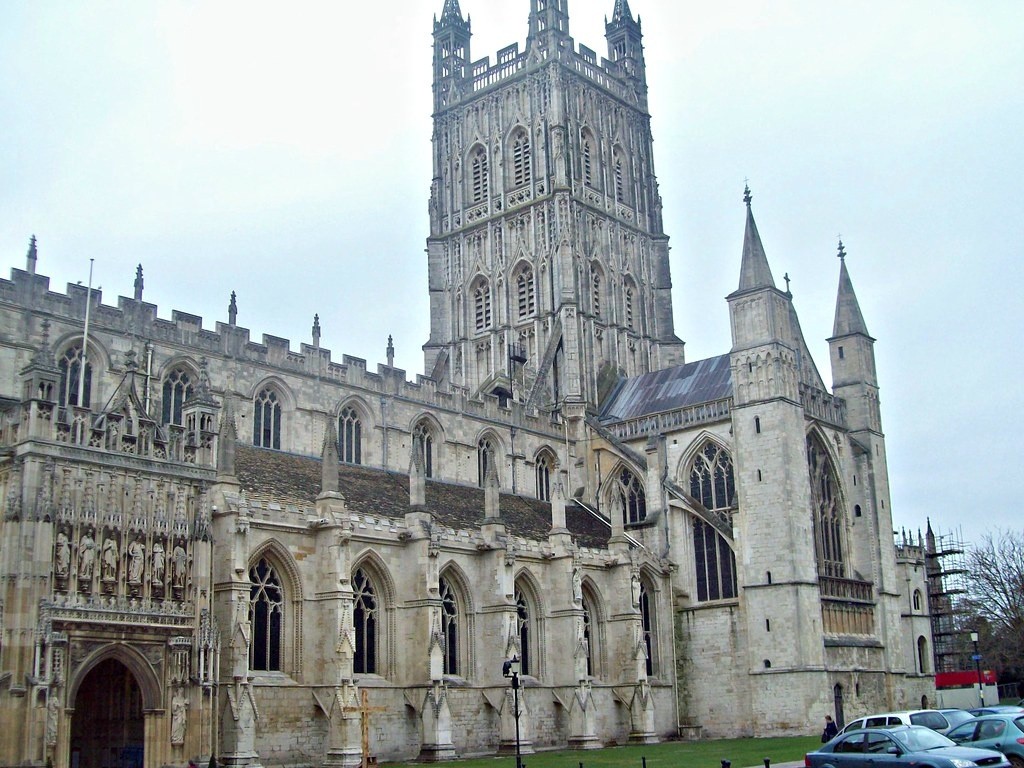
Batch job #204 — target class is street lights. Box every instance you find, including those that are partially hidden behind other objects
[970,627,985,708]
[502,654,523,768]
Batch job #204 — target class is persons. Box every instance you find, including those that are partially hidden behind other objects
[45,528,191,746]
[823,715,838,742]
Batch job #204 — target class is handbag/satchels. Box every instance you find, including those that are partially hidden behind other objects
[821,729,829,743]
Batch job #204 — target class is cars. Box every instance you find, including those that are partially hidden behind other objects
[805,723,1012,768]
[945,713,1024,768]
[830,705,1024,743]
